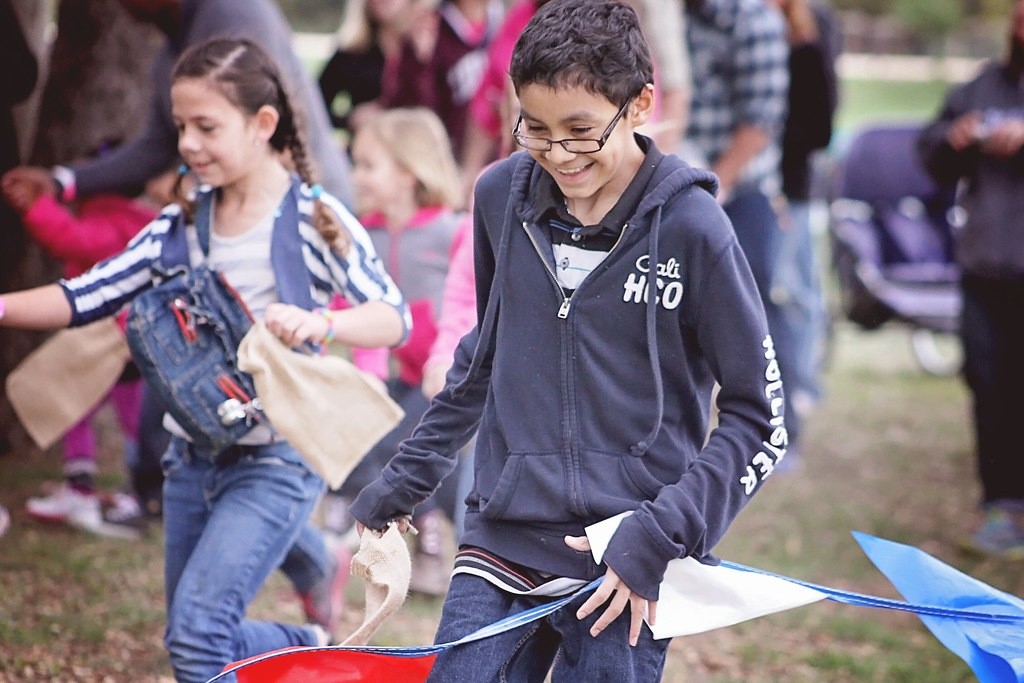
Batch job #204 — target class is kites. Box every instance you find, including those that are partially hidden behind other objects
[206,529,1024,683]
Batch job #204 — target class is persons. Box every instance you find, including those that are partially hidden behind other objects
[313,1,436,127]
[773,2,840,416]
[679,2,800,469]
[6,137,162,525]
[396,3,517,156]
[348,1,789,683]
[321,108,475,595]
[1,38,412,682]
[463,1,692,205]
[912,0,1024,559]
[2,1,348,540]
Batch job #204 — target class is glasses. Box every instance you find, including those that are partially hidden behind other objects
[512,94,635,153]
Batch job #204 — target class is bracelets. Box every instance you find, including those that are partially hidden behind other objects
[309,308,335,348]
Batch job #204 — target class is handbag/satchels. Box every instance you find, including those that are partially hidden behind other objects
[125,260,267,465]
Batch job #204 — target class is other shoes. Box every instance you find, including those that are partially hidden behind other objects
[22,473,163,544]
[405,508,456,601]
[954,497,1024,561]
